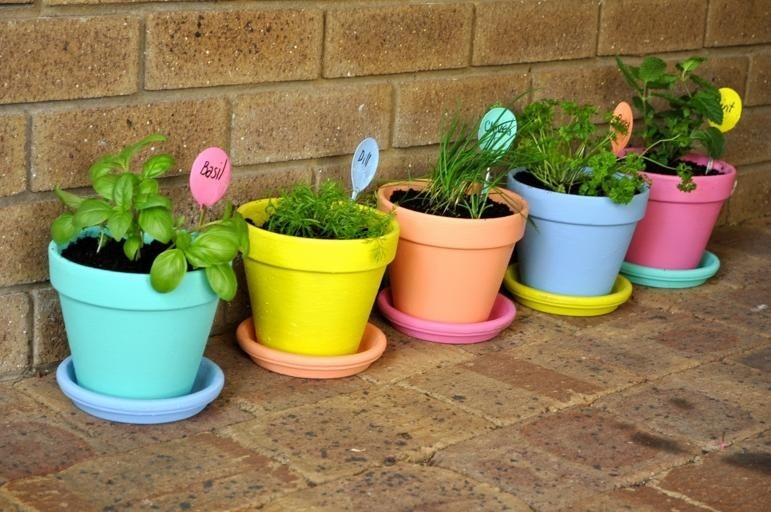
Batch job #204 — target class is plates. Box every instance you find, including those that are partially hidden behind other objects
[378,288,517,345]
[55,356,225,425]
[236,318,387,380]
[503,262,634,318]
[621,250,723,291]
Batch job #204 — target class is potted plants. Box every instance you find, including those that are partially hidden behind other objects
[44,129,253,397]
[377,85,558,322]
[485,92,699,296]
[235,169,402,361]
[611,54,741,272]
